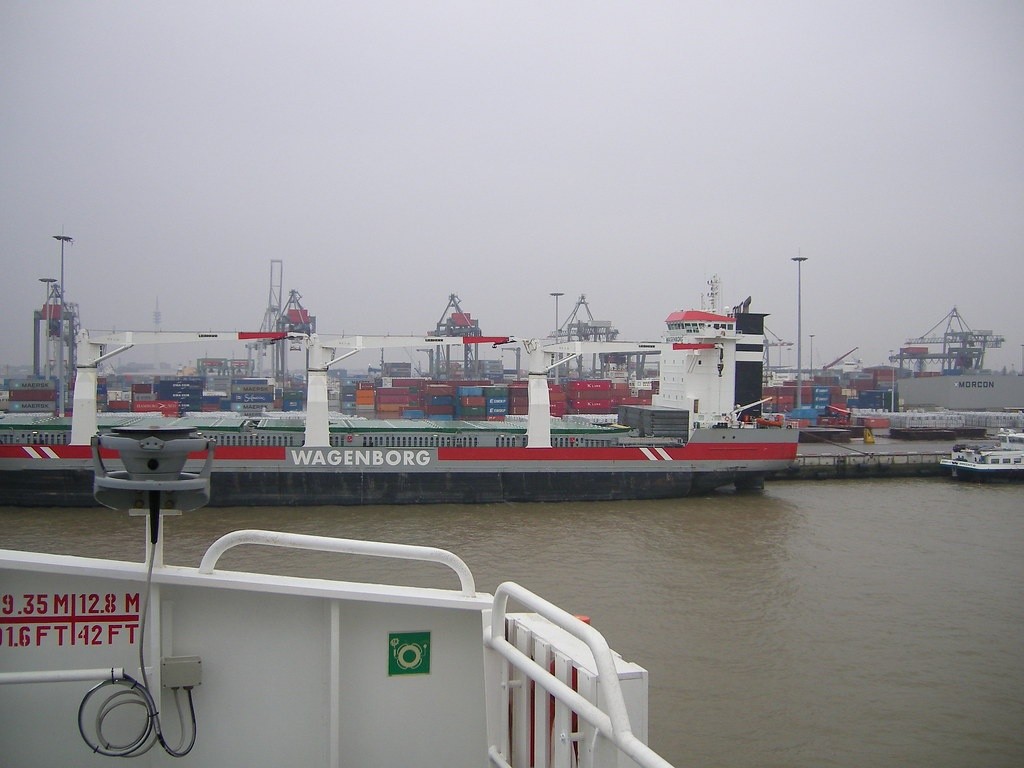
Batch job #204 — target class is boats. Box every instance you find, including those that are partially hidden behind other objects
[940,427,1024,484]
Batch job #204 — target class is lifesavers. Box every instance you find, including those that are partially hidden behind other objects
[347,435,353,442]
[725,416,730,422]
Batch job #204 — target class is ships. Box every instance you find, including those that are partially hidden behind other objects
[0,327,799,510]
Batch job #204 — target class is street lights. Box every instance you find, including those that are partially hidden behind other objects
[50,224,76,417]
[790,257,809,410]
[549,292,564,387]
[38,278,60,382]
